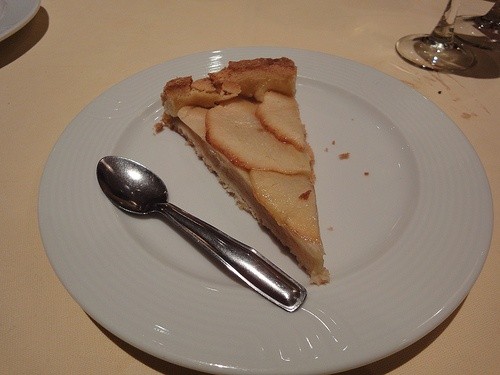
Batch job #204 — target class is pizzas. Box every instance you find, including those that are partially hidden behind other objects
[154,57,330,286]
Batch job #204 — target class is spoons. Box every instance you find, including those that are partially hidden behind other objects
[96,156,308,312]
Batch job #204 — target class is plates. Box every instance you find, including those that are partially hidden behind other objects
[37,46,493,375]
[0,0,41,40]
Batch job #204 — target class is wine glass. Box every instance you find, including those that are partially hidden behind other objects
[454,0,500,49]
[395,0,474,71]
